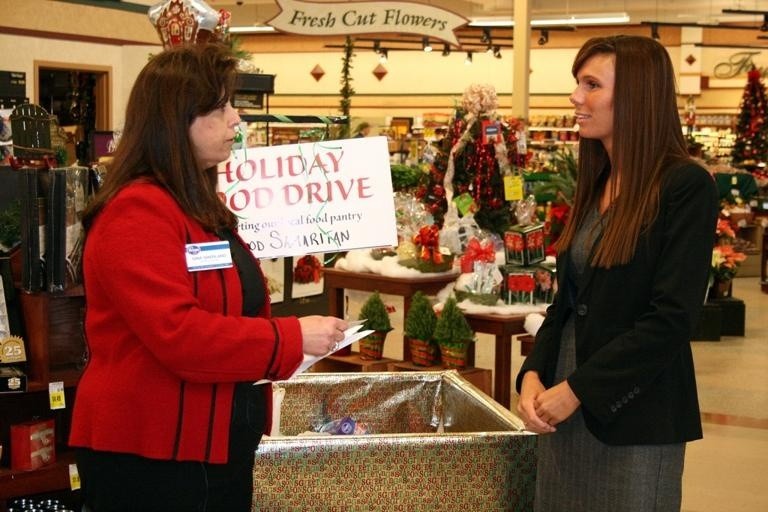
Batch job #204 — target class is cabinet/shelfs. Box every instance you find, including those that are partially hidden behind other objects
[241,113,738,174]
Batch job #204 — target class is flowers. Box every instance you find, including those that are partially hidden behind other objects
[717,244,745,280]
[717,216,738,240]
[711,248,725,269]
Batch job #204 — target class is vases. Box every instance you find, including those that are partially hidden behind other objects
[715,278,731,297]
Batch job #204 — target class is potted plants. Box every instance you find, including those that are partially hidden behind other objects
[432,294,479,370]
[356,291,393,360]
[403,291,437,367]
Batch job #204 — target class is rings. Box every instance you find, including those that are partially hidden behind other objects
[329,341,339,351]
[524,422,528,426]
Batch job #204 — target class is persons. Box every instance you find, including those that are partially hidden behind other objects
[515,35,721,512]
[66,41,348,512]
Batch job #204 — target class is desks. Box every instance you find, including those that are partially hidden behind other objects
[252,374,541,511]
[313,264,460,374]
[465,314,546,412]
